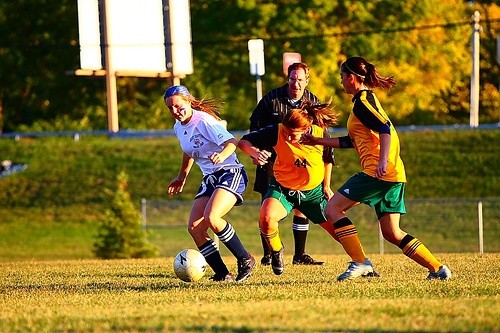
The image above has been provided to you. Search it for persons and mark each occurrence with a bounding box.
[248,61,321,266]
[164,86,256,282]
[299,56,450,280]
[239,96,342,275]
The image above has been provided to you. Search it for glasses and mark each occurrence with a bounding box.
[288,78,305,84]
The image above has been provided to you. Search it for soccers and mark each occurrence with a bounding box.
[174,249,207,281]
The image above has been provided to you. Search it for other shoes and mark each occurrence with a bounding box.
[261,254,272,265]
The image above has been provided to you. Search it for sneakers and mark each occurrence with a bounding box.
[293,254,325,265]
[209,272,236,283]
[337,257,374,281]
[425,264,452,279]
[269,241,285,275]
[235,252,255,282]
